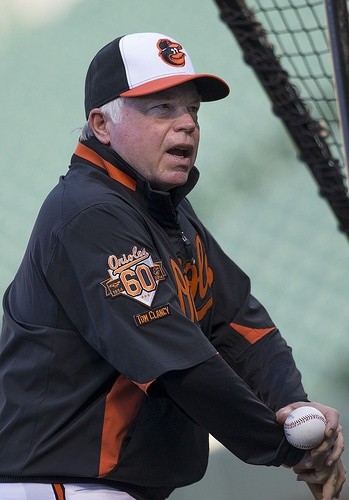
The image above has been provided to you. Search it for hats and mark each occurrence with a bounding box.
[84,33,230,121]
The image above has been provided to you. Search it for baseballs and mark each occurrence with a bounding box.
[284,407,326,449]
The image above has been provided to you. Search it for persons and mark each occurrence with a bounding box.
[0,27,348,500]
